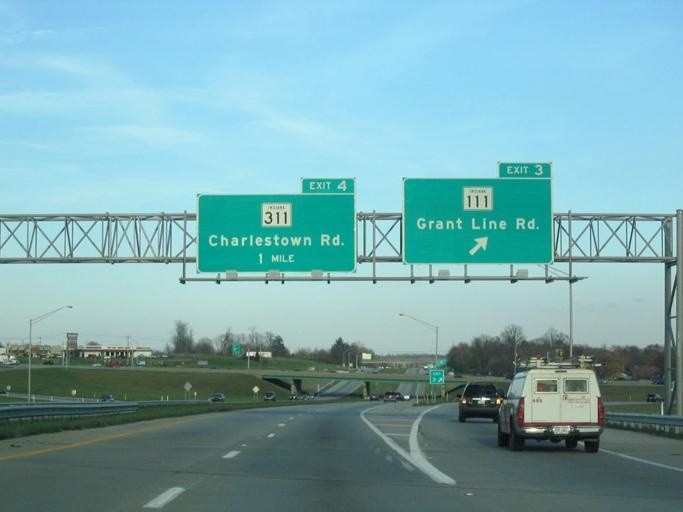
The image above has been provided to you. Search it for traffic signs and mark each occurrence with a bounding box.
[430,369,444,385]
[401,176,554,265]
[195,193,357,273]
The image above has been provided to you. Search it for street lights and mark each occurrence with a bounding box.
[341,349,372,370]
[398,313,438,402]
[27,305,72,403]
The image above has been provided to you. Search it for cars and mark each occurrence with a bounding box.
[647,393,663,402]
[264,391,275,400]
[207,391,224,401]
[96,394,113,403]
[447,369,461,376]
[288,392,314,400]
[364,392,410,401]
[652,376,664,384]
[90,358,146,368]
[494,388,506,399]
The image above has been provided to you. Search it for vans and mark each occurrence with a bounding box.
[493,348,604,453]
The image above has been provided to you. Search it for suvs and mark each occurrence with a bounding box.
[455,381,504,422]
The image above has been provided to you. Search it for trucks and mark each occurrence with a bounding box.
[0,348,19,365]
